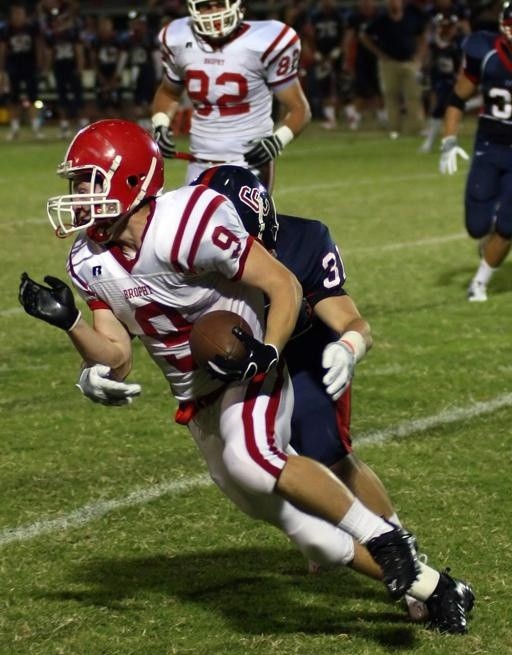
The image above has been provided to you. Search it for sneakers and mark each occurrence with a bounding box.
[429,566,477,636]
[469,278,490,302]
[367,517,420,601]
[406,594,428,621]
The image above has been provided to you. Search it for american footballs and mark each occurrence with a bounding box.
[188,310,253,375]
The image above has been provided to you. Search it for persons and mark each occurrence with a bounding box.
[87,13,128,118]
[0,8,46,141]
[362,1,433,140]
[187,165,404,538]
[150,0,313,185]
[440,1,512,303]
[42,5,89,140]
[418,11,468,154]
[120,11,161,119]
[284,0,362,133]
[19,119,474,633]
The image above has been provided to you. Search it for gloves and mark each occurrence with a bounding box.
[151,113,176,158]
[18,273,81,334]
[207,328,278,382]
[76,366,142,407]
[322,333,366,403]
[440,137,468,176]
[244,126,293,166]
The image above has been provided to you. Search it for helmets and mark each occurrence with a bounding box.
[189,0,243,39]
[189,165,279,258]
[498,3,512,40]
[46,119,164,237]
